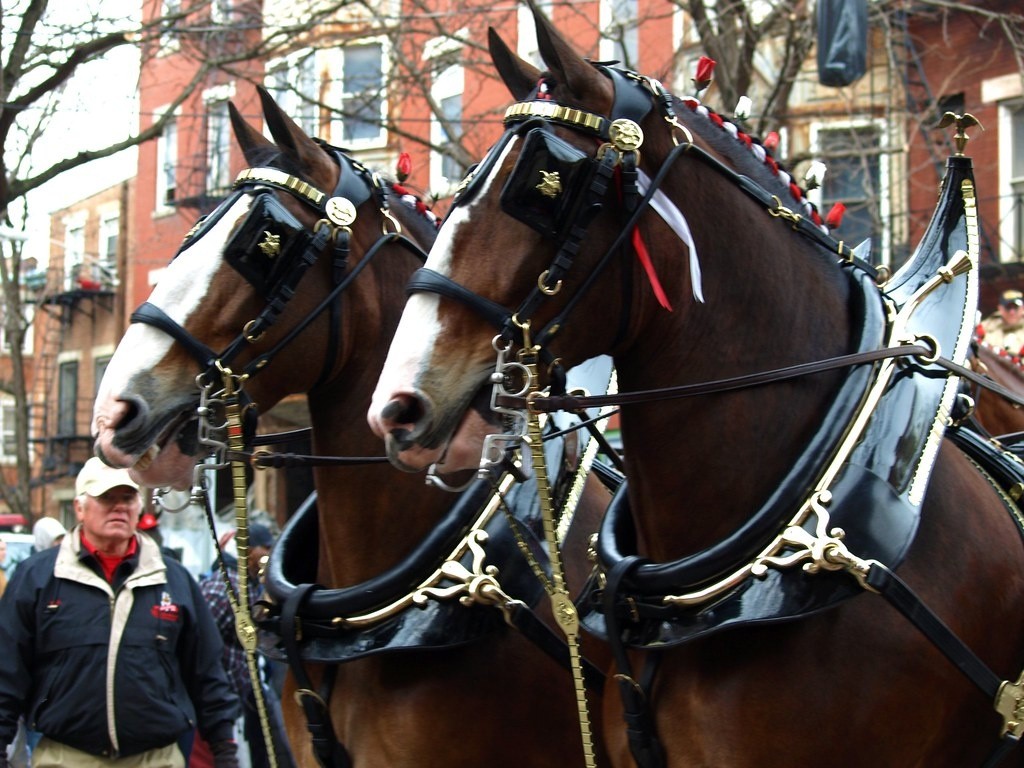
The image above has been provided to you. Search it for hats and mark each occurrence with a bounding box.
[248,524,275,548]
[76,456,140,497]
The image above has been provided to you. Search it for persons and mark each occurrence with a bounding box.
[974,290,1024,366]
[0,455,276,768]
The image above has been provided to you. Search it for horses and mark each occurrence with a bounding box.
[91,1,1024,768]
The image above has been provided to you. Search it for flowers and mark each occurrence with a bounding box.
[383,153,444,230]
[674,55,848,238]
